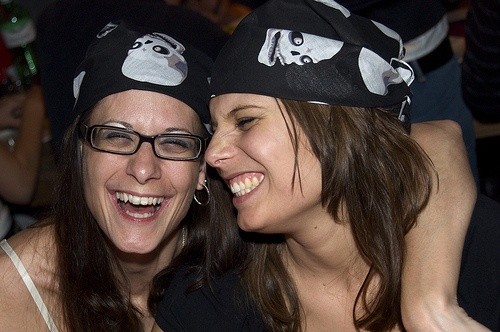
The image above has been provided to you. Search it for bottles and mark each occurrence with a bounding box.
[0,0,42,85]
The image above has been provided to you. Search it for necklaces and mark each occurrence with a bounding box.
[181,226,185,248]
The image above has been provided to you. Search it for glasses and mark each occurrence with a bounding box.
[80,122,203,161]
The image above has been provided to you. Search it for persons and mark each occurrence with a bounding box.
[0,0,500,332]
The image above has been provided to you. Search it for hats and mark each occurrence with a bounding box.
[73,18,213,135]
[207,0,415,135]
